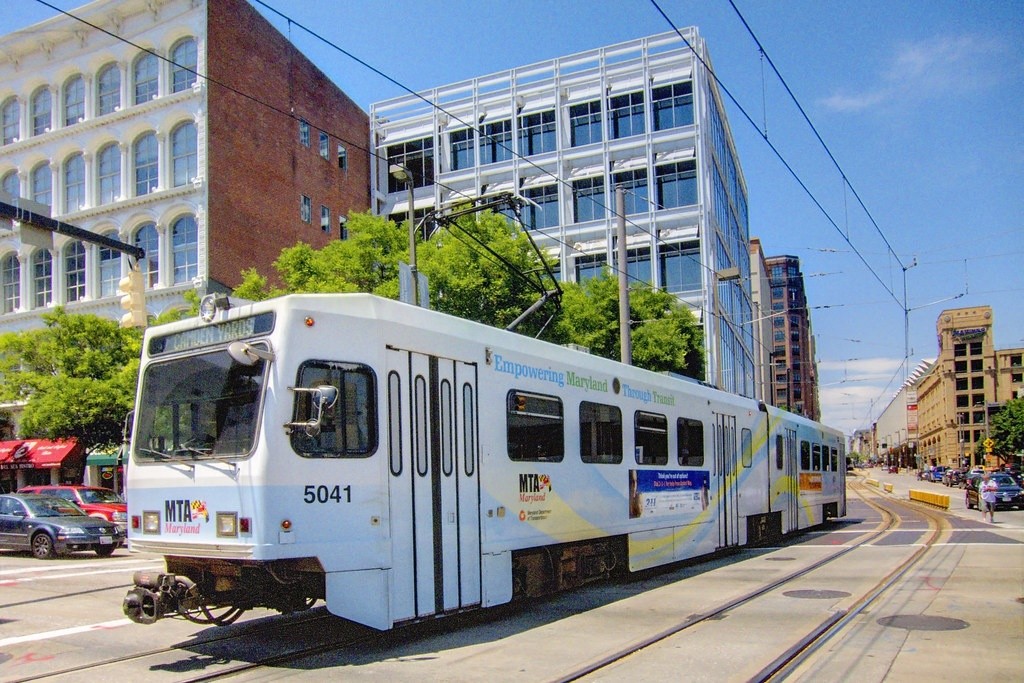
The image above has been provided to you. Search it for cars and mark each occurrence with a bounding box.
[966,473,1024,511]
[943,469,986,487]
[917,470,930,481]
[882,465,888,471]
[0,493,126,559]
[1006,470,1024,487]
[889,466,898,474]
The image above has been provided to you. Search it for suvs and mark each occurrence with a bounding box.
[17,486,127,538]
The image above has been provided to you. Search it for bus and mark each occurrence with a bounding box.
[121,290,847,633]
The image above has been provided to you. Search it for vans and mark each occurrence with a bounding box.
[929,466,951,482]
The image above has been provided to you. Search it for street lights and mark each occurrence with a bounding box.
[391,164,418,302]
[974,405,985,437]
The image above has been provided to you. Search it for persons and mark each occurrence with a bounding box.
[978,472,999,523]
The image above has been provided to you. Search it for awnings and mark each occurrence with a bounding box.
[85,448,122,466]
[0,436,79,471]
